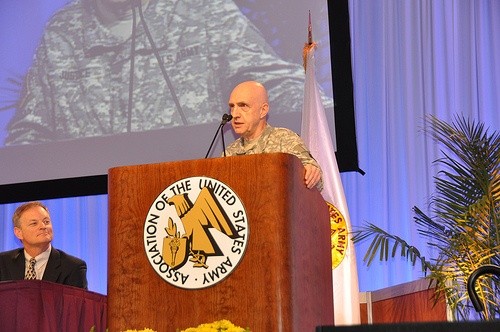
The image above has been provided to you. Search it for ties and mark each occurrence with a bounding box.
[24,257,36,280]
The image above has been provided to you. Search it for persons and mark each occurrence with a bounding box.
[3,0,336,150]
[222,81,323,192]
[0,202,88,290]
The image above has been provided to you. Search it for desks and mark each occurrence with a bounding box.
[0,280,107,332]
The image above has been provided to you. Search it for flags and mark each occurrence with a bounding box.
[300,41,360,326]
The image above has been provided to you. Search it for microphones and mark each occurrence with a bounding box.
[221,115,233,157]
[205,114,228,159]
